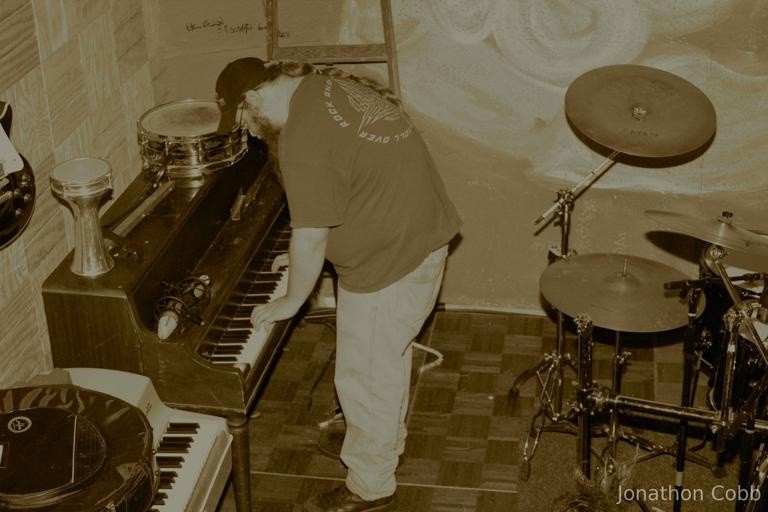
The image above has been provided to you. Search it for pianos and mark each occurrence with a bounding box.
[1,368,233,512]
[40,130,292,512]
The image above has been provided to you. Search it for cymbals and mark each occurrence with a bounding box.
[643,209,768,257]
[565,64,716,159]
[539,252,707,333]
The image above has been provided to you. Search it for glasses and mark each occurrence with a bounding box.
[237,100,250,134]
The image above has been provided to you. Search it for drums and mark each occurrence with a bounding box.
[683,262,768,377]
[136,98,248,183]
[49,156,115,279]
[710,298,768,434]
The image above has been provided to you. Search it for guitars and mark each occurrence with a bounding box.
[0,102,35,249]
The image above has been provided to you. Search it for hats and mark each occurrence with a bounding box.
[215,57,266,138]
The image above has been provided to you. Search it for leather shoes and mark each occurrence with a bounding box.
[315,430,348,460]
[302,482,398,511]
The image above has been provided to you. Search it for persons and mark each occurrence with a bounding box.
[214,56,463,511]
